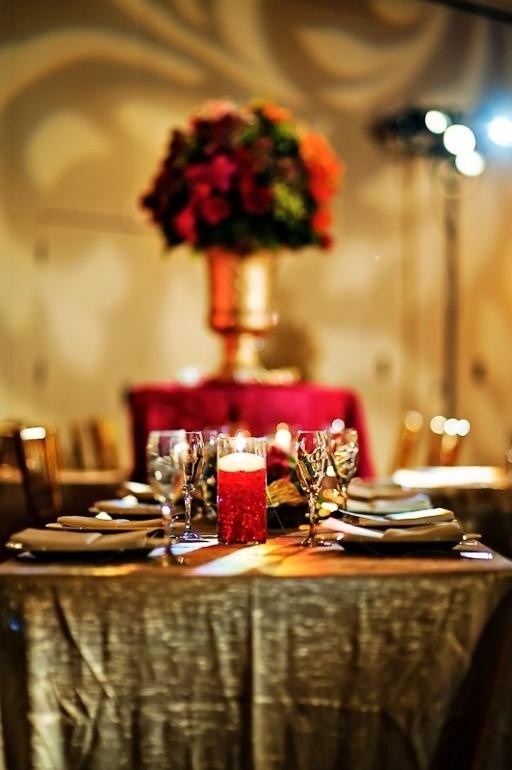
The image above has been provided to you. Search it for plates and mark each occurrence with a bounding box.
[8,481,174,563]
[327,494,465,552]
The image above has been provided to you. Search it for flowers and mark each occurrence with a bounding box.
[137,95,336,258]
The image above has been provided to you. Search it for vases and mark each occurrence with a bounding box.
[198,243,300,386]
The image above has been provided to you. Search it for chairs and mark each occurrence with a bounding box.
[12,425,64,522]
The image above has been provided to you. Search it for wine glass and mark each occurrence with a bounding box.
[174,431,206,541]
[327,428,359,511]
[294,428,333,546]
[144,428,191,568]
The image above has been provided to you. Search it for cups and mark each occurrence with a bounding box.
[216,435,270,544]
